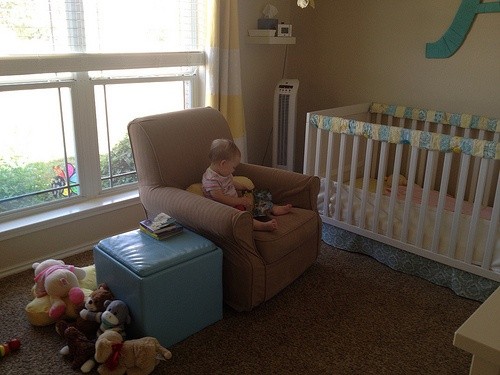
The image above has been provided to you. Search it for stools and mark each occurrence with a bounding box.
[93,227,223,347]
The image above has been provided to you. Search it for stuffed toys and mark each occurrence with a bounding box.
[25,259,172,375]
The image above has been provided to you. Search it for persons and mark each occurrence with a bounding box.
[201,139,291,231]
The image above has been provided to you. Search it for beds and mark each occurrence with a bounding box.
[304,104,500,303]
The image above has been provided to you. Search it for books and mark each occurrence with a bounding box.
[140,212,184,242]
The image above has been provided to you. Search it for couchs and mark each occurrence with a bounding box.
[127,106,322,310]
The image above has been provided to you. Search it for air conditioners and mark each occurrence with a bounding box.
[273,78,299,171]
